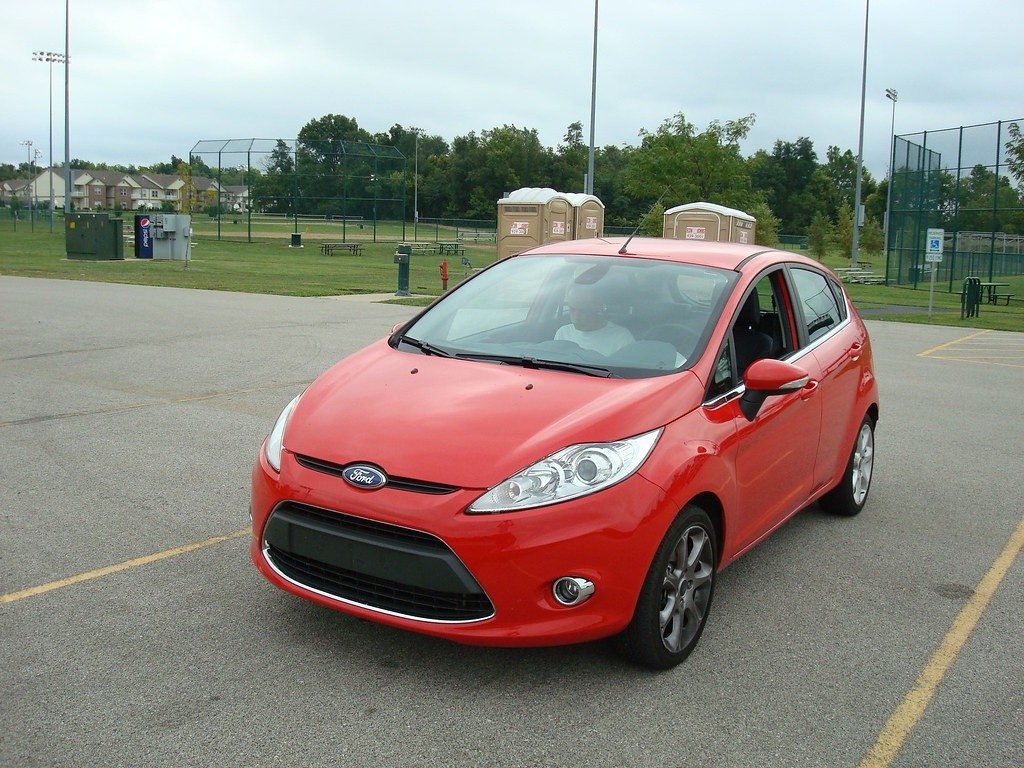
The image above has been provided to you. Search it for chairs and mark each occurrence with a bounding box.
[711,282,776,382]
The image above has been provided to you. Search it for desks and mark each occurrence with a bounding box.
[962,282,1010,304]
[321,243,362,255]
[436,242,463,254]
[404,242,432,255]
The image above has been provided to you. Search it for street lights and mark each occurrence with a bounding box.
[881,86,898,257]
[22,139,33,211]
[34,148,43,204]
[31,51,66,215]
[409,126,427,228]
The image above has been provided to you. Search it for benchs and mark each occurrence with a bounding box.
[834,268,863,280]
[394,247,423,253]
[991,294,1015,306]
[866,279,897,285]
[846,272,874,284]
[320,245,354,251]
[956,291,983,302]
[327,249,365,256]
[446,249,466,256]
[856,275,885,284]
[411,249,436,255]
[431,246,453,254]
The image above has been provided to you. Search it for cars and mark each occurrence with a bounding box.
[244,234,880,671]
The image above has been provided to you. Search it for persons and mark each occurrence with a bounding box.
[553,283,636,357]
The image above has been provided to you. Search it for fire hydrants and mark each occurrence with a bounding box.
[440,258,448,289]
